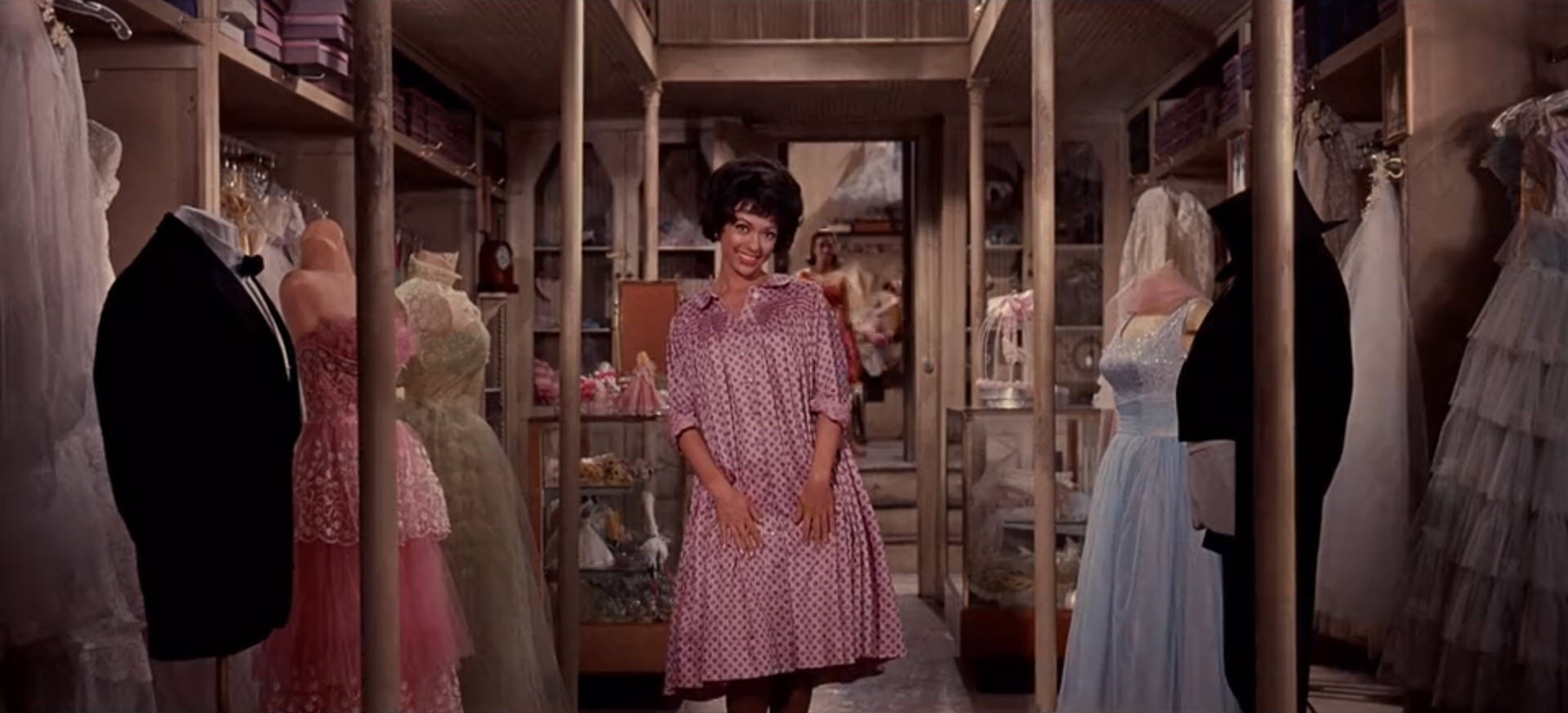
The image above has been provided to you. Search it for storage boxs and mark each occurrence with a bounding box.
[217,0,479,166]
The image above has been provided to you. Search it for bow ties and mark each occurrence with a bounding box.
[237,255,265,277]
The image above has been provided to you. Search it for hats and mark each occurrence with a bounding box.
[1207,171,1352,281]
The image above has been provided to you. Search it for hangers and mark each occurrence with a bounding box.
[222,131,298,212]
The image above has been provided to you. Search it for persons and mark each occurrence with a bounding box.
[251,219,470,713]
[1053,267,1236,713]
[791,229,873,458]
[658,156,905,713]
[391,250,572,713]
[92,205,304,662]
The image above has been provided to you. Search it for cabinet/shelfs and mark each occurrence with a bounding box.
[78,0,504,713]
[531,412,704,673]
[508,117,735,394]
[945,0,1568,713]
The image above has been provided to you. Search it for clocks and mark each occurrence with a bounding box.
[478,240,518,293]
